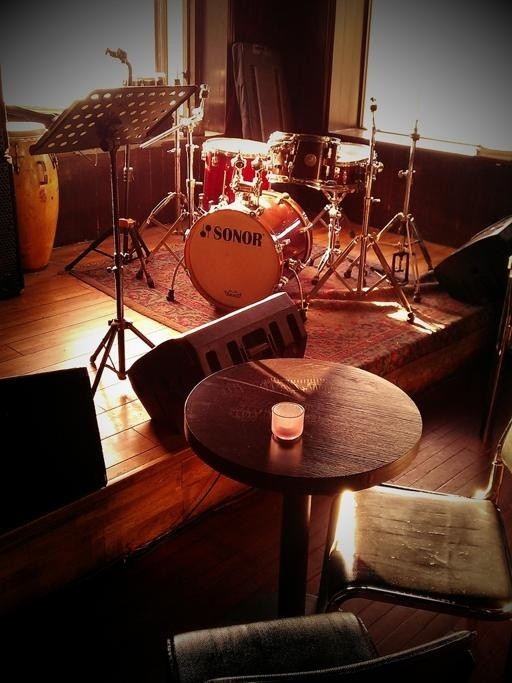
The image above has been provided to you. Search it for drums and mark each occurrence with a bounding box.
[199,137,271,216]
[7,127,60,272]
[183,188,312,314]
[262,130,340,191]
[334,142,383,196]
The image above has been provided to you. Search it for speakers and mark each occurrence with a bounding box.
[0,367,108,536]
[419,215,512,306]
[128,292,307,434]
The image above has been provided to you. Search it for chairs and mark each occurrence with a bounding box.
[166,609,476,678]
[317,417,512,620]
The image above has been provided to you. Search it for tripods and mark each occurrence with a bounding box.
[343,119,433,286]
[305,97,415,324]
[90,149,155,400]
[309,190,368,285]
[130,72,208,302]
[64,45,155,289]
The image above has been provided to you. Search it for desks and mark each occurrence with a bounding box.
[183,356,425,618]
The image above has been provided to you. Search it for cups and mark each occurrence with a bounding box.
[272,401,305,439]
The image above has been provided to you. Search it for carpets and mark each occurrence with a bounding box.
[67,229,481,380]
